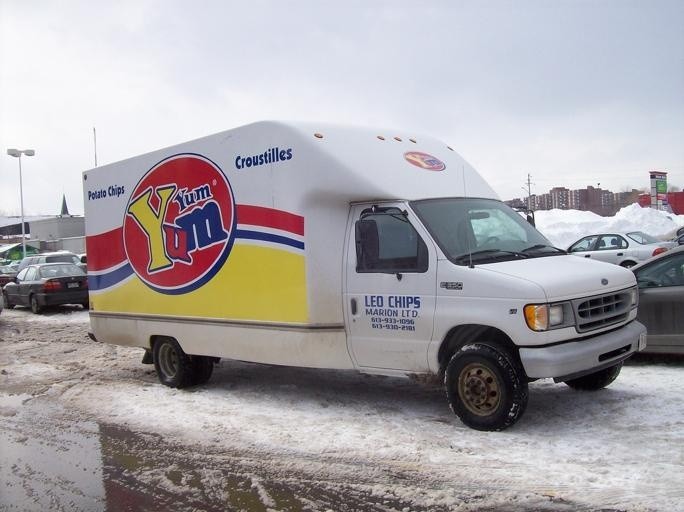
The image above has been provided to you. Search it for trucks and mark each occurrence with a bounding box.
[82,120,647,432]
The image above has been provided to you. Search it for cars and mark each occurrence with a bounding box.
[0,250,89,313]
[664,226,684,245]
[627,245,683,357]
[563,229,679,267]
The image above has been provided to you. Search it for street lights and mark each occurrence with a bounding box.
[521,186,536,214]
[6,148,37,259]
